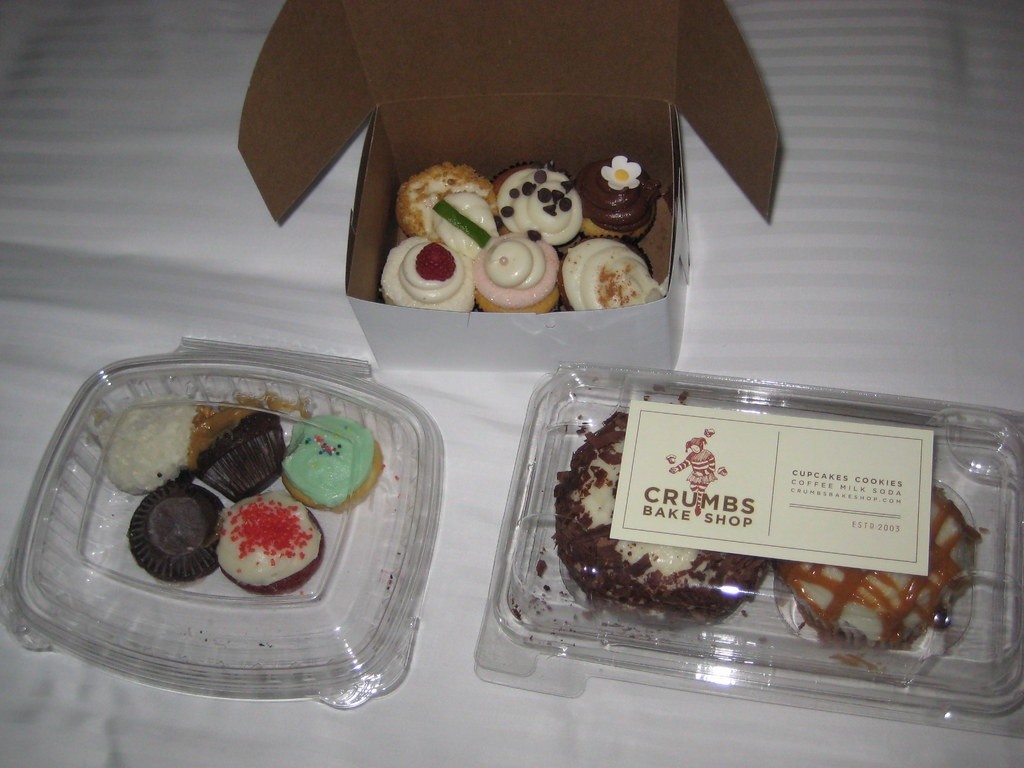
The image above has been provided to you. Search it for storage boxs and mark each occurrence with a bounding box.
[237,0,781,372]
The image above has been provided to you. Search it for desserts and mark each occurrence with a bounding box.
[381,156,662,312]
[553,409,983,650]
[106,401,383,594]
